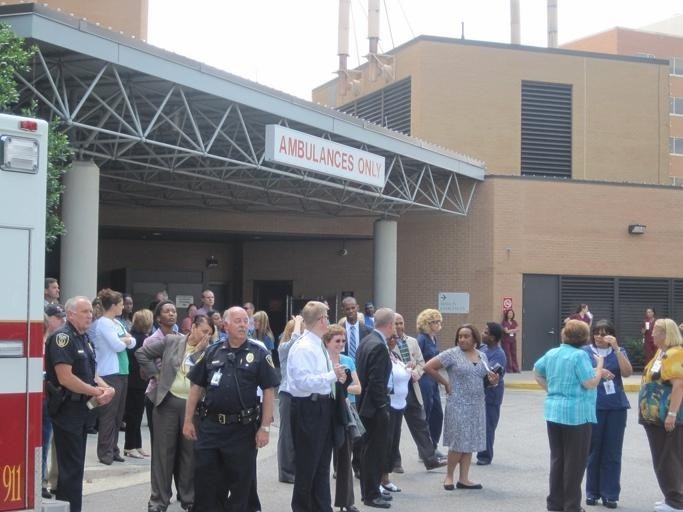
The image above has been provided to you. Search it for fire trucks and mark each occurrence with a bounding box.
[1,113,49,512]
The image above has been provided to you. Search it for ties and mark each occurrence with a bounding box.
[321,341,336,400]
[348,326,356,362]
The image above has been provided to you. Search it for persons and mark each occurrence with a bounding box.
[563,302,592,345]
[639,307,658,367]
[474,324,508,465]
[531,320,603,511]
[677,321,682,330]
[41,278,443,511]
[423,323,499,490]
[637,318,683,511]
[501,309,520,373]
[580,318,633,507]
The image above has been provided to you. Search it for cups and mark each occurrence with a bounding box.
[586,312,593,319]
[644,322,649,330]
[86,395,100,409]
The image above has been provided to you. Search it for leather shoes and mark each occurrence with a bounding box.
[100,448,150,465]
[418,454,491,490]
[333,467,404,508]
[548,497,683,512]
[42,488,51,498]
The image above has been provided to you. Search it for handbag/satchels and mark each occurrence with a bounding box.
[484,362,505,388]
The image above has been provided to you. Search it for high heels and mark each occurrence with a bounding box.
[341,505,360,512]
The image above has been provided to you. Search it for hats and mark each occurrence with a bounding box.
[45,303,66,317]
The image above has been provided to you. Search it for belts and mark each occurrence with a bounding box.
[206,410,252,425]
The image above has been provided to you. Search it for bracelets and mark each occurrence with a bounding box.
[667,411,677,416]
[614,348,621,355]
